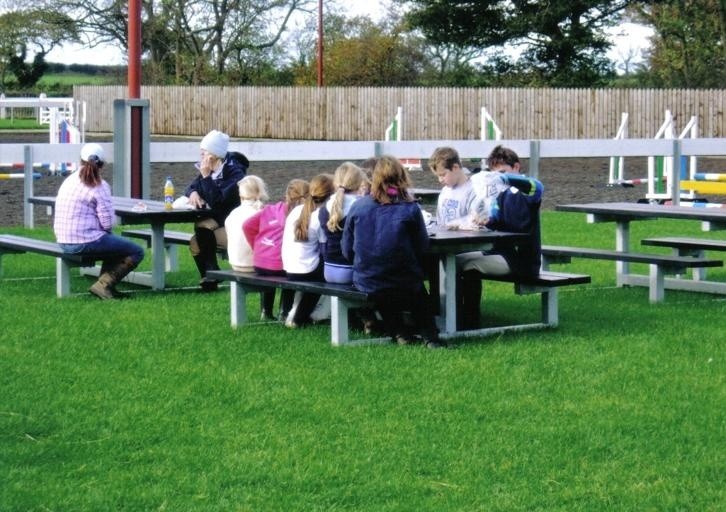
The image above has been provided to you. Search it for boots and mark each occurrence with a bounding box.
[89,256,136,298]
[192,227,223,292]
[462,269,482,329]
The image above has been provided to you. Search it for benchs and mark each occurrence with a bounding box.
[538,244,723,303]
[0,233,95,298]
[121,229,228,273]
[488,269,591,327]
[204,270,408,347]
[639,238,725,282]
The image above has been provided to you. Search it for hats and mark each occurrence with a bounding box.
[200,130,229,158]
[80,143,104,162]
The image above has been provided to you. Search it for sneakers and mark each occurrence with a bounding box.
[262,311,440,347]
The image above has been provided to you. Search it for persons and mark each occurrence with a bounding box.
[183,129,249,292]
[53,142,145,301]
[224,145,545,349]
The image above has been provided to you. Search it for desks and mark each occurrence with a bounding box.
[555,202,726,298]
[28,195,217,292]
[331,224,559,348]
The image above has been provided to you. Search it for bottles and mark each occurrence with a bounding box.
[163,176,174,210]
